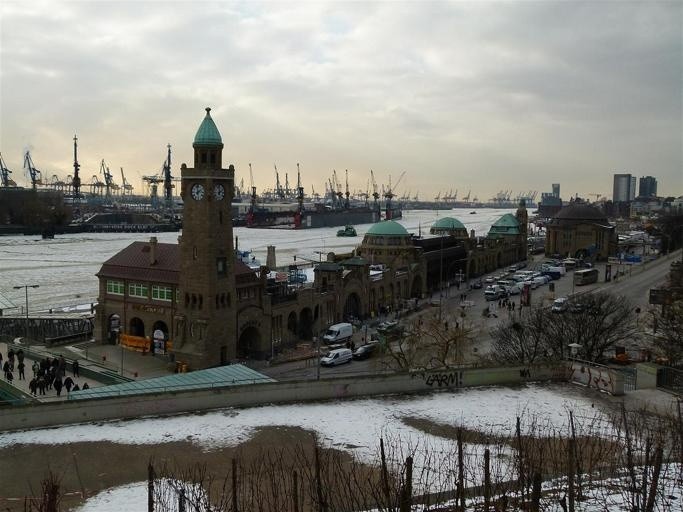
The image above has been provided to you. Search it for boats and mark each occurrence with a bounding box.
[336,222,357,237]
[468,210,477,214]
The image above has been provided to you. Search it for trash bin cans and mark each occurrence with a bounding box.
[177,361,189,373]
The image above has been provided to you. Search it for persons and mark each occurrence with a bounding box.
[460,292,464,304]
[464,292,467,302]
[498,296,515,311]
[0,348,89,395]
[369,303,392,320]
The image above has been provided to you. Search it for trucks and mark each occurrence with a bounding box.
[549,297,569,314]
[320,320,404,368]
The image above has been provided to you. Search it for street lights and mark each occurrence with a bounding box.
[312,291,328,380]
[566,342,582,390]
[313,250,329,293]
[13,284,39,354]
[78,313,92,363]
[108,313,124,377]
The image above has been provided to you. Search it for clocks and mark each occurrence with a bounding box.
[212,183,226,203]
[189,183,203,201]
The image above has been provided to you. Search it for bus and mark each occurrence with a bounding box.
[572,269,600,285]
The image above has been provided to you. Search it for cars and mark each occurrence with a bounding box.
[469,255,581,302]
